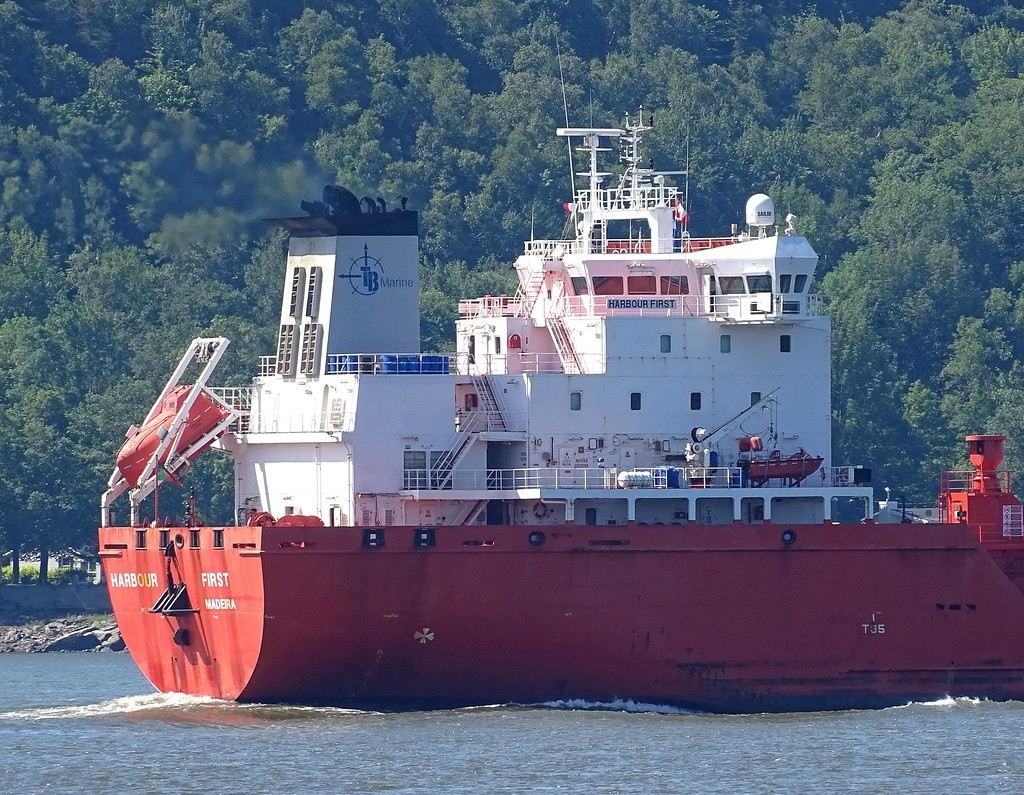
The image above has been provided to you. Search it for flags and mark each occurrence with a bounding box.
[564,203,577,216]
[675,200,691,224]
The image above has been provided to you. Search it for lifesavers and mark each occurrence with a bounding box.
[533,502,548,518]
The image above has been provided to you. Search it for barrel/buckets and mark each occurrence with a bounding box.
[422,356,430,374]
[710,450,718,467]
[339,357,347,374]
[383,356,397,374]
[671,229,681,252]
[350,356,358,374]
[484,295,491,309]
[444,357,449,374]
[397,356,407,374]
[654,467,680,489]
[431,356,438,374]
[407,356,420,374]
[329,356,337,374]
[465,394,477,410]
[499,293,508,309]
[733,473,741,488]
[439,357,443,374]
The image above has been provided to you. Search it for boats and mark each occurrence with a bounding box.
[738,450,824,482]
[98,42,1024,713]
